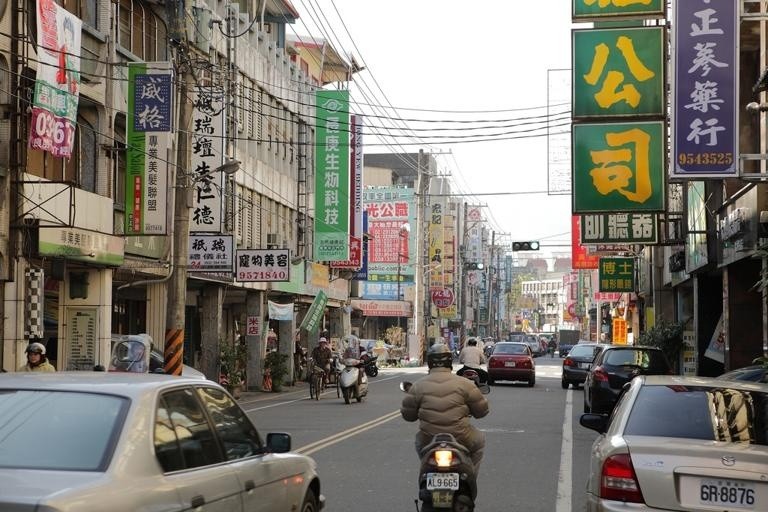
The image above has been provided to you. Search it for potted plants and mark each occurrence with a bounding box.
[216,334,247,399]
[264,352,289,392]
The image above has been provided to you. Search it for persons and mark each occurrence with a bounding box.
[117,331,158,372]
[311,334,332,388]
[454,337,490,388]
[342,339,368,386]
[547,337,557,359]
[16,341,57,373]
[383,335,398,365]
[399,341,491,479]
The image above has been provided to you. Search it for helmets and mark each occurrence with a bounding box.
[468,339,477,344]
[429,343,452,368]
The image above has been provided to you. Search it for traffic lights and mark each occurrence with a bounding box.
[511,240,540,251]
[463,261,483,271]
[546,302,555,306]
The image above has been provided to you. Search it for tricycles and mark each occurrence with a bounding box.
[307,357,342,401]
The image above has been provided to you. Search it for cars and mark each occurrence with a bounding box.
[504,329,556,356]
[0,373,325,512]
[579,375,767,512]
[485,341,538,387]
[359,338,400,363]
[560,343,603,389]
[714,365,768,383]
[582,345,673,418]
[110,332,209,382]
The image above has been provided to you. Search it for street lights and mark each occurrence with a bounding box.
[162,156,243,377]
[397,224,411,302]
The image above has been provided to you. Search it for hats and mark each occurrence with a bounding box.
[318,337,327,343]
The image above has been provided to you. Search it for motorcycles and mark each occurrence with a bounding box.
[358,347,380,377]
[337,353,369,404]
[483,344,492,358]
[397,379,490,511]
[455,349,487,391]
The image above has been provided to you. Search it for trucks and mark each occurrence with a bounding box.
[557,329,580,358]
